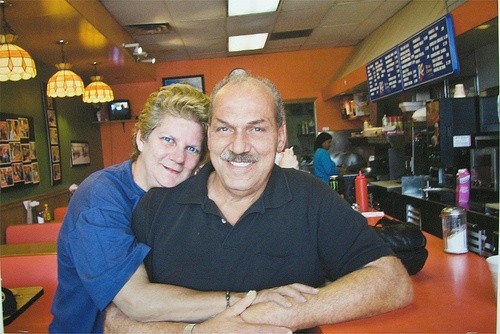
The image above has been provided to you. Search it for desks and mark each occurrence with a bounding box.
[316,209,496,334]
[3,286,44,326]
[0,242,57,257]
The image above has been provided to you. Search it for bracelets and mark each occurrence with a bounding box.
[225,291,231,309]
[182,324,197,334]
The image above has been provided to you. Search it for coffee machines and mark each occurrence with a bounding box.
[43,203,51,222]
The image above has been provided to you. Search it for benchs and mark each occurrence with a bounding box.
[54,207,67,220]
[0,255,58,334]
[6,224,63,245]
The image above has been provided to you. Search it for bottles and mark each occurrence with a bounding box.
[343,100,349,118]
[453,84,467,98]
[383,113,404,130]
[363,120,369,130]
[297,119,315,136]
[486,255,498,296]
[354,170,369,212]
[439,207,467,253]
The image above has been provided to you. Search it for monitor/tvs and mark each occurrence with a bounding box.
[108,99,132,119]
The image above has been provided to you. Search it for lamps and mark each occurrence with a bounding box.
[47,39,84,97]
[126,43,155,64]
[0,0,37,81]
[83,62,114,103]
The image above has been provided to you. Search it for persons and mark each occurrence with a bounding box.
[312,132,346,188]
[104,73,414,334]
[47,82,318,334]
